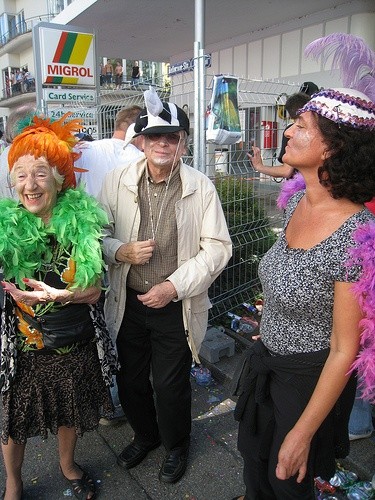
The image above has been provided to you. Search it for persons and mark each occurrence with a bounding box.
[130,61,142,91]
[0,111,115,500]
[115,61,123,91]
[98,59,113,88]
[0,104,143,427]
[230,87,375,500]
[5,68,35,98]
[246,81,375,440]
[95,85,232,485]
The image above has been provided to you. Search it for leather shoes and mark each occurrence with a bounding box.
[159,447,189,484]
[117,439,157,469]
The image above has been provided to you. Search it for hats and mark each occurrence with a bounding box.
[284,81,320,118]
[295,33,375,131]
[8,111,89,189]
[134,85,190,135]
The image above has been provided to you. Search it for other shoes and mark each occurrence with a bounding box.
[231,495,245,500]
[99,415,127,425]
[349,426,373,440]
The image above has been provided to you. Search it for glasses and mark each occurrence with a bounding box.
[148,132,180,144]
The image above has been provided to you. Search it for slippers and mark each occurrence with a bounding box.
[1,480,23,500]
[60,462,96,500]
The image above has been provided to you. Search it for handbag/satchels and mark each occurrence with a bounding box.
[205,75,242,145]
[39,303,96,348]
